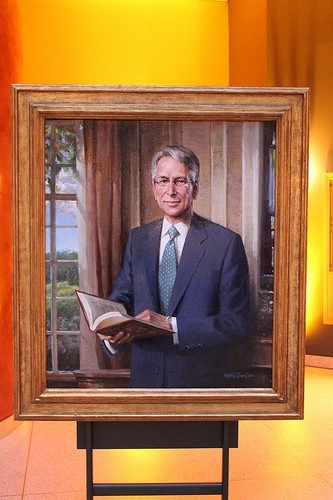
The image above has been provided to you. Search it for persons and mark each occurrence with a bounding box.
[97,144,250,388]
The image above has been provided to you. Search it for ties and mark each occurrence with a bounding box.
[158,226,179,312]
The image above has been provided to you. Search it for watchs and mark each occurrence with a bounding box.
[167,315,173,329]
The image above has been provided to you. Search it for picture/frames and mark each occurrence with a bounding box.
[9,84,311,422]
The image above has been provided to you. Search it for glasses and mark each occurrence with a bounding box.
[155,177,193,187]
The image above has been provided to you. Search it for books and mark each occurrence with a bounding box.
[75,288,174,343]
